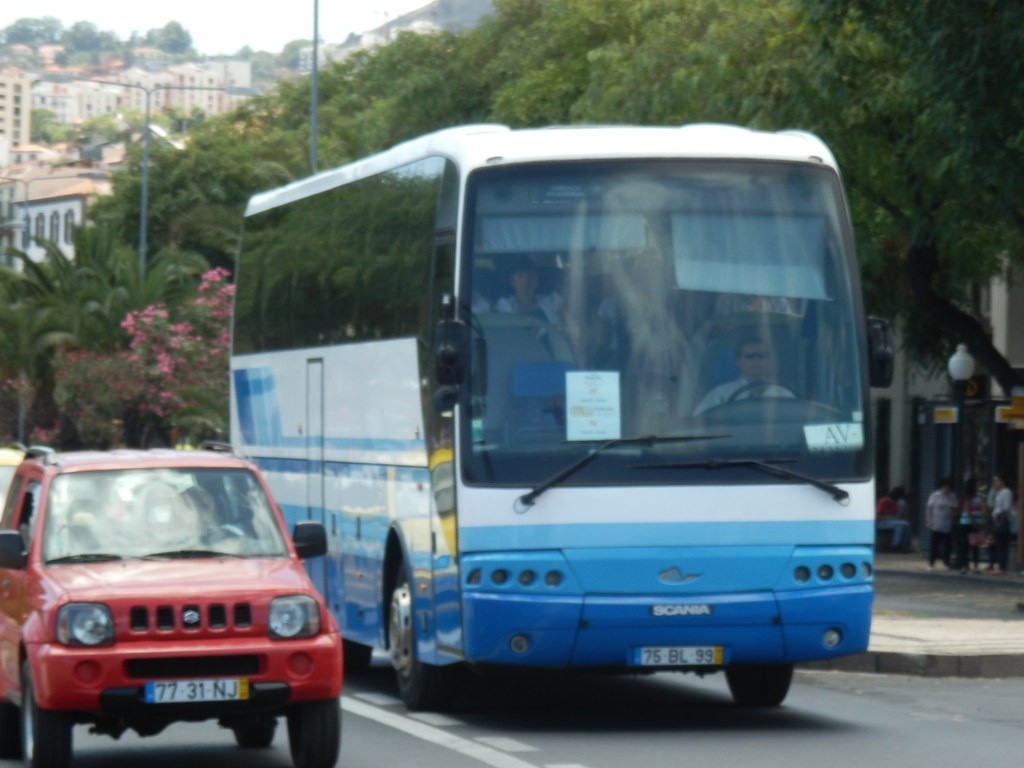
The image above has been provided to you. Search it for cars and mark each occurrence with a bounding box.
[1,442,29,523]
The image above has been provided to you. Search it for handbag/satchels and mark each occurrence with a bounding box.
[969,533,988,545]
[996,511,1017,541]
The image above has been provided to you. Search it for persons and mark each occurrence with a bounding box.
[879,470,1019,576]
[456,254,798,426]
[53,482,242,557]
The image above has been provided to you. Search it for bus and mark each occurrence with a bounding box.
[224,123,892,714]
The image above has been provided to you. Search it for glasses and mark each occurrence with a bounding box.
[739,351,765,358]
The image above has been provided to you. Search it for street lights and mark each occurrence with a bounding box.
[37,69,263,294]
[941,342,977,569]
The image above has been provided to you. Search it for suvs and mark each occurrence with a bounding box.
[0,444,344,768]
